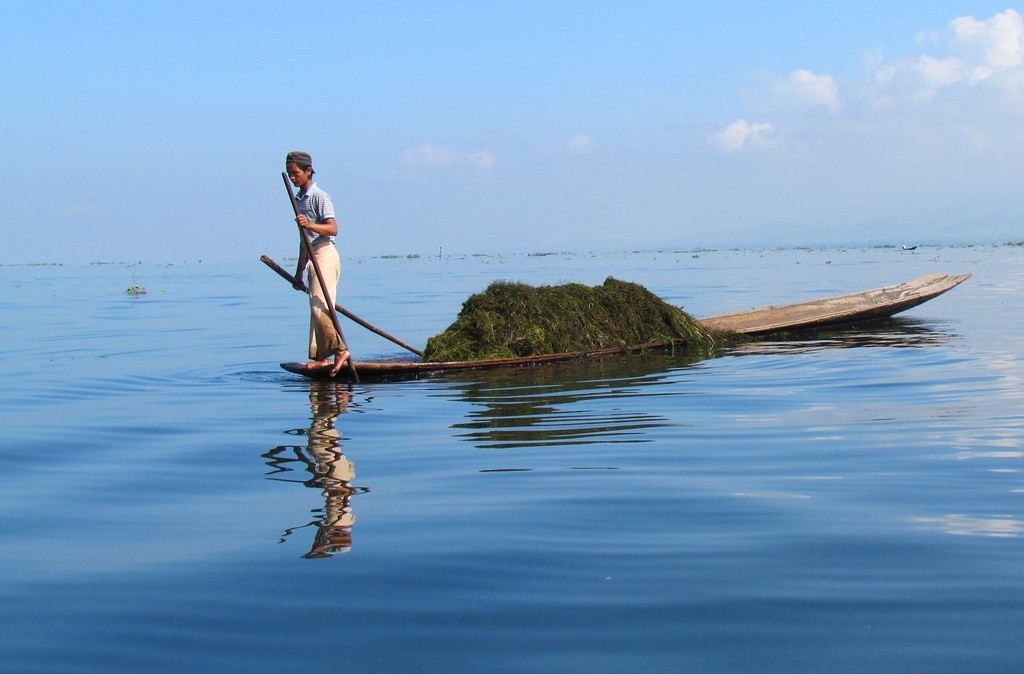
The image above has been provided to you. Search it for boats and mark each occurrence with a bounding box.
[901,244,917,250]
[278,270,973,383]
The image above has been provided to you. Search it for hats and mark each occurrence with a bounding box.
[286,151,315,174]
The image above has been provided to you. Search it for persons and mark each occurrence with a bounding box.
[286,152,349,377]
